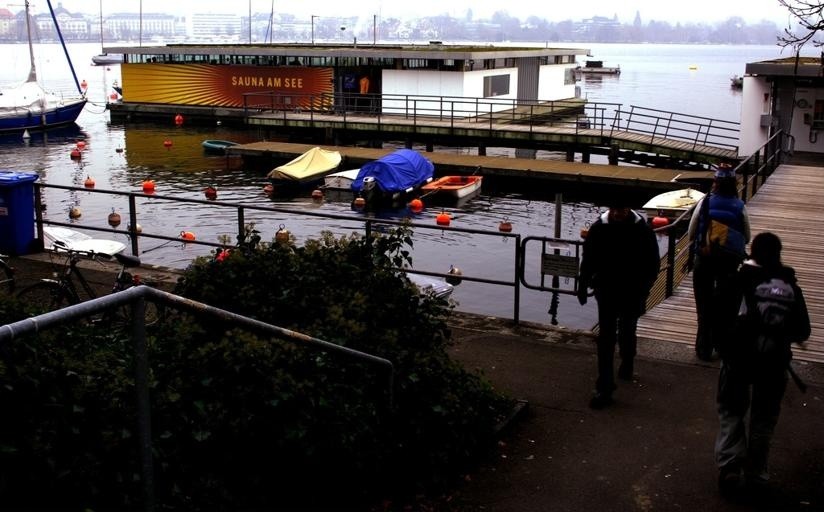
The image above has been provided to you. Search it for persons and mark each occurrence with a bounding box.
[686,163,751,362]
[577,187,661,409]
[703,231,812,504]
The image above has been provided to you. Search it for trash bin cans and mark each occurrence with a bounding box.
[0,170,40,257]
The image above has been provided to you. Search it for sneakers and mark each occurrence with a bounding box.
[695,349,722,361]
[588,359,634,410]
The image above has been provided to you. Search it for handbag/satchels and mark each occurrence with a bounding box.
[696,218,746,262]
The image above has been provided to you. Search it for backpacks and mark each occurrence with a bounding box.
[742,267,791,352]
